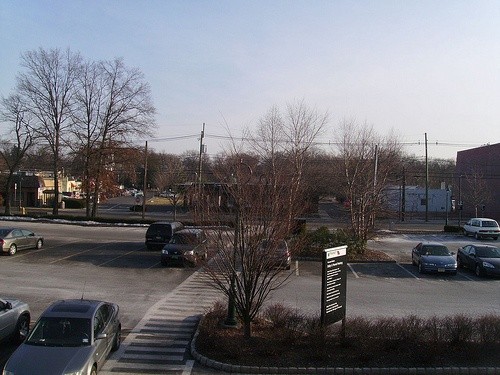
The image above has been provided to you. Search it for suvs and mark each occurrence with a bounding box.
[258,238,291,270]
[161,228,210,268]
[145,222,186,248]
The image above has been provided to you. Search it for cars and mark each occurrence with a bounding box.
[0,298,31,343]
[412,242,457,276]
[127,188,179,198]
[463,218,500,240]
[0,227,44,256]
[2,299,122,375]
[457,244,500,278]
